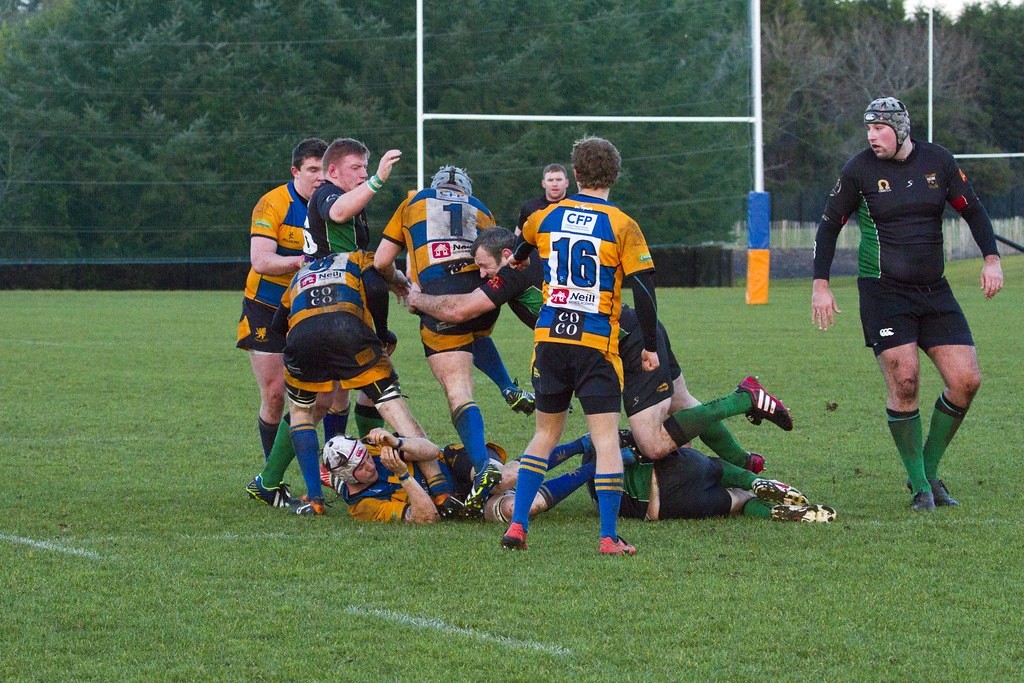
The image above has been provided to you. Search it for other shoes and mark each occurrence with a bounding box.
[769,505,836,525]
[753,478,809,506]
[585,431,633,447]
[631,445,656,463]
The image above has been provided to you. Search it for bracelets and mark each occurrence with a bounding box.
[397,472,413,485]
[397,438,405,448]
[296,255,305,269]
[366,174,386,192]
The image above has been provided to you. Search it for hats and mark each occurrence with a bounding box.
[323,434,367,486]
[864,97,910,146]
[430,164,473,196]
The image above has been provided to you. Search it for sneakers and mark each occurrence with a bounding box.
[290,496,326,517]
[744,452,767,474]
[437,493,463,519]
[502,522,529,550]
[503,379,536,417]
[246,474,301,509]
[907,479,956,505]
[320,462,332,488]
[911,489,935,511]
[599,536,636,556]
[464,460,502,518]
[735,375,793,432]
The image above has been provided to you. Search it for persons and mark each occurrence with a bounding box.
[500,136,660,554]
[236,137,837,524]
[810,96,1003,511]
